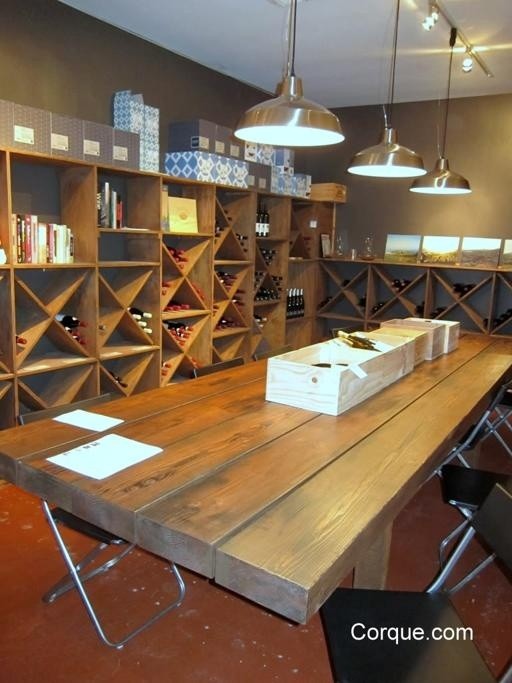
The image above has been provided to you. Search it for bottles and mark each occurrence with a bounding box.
[254,271,284,302]
[97,323,108,333]
[108,371,129,389]
[254,314,269,329]
[161,205,251,381]
[358,232,376,259]
[334,329,386,353]
[14,334,27,346]
[127,307,153,337]
[256,201,271,238]
[56,306,90,349]
[303,235,312,251]
[259,248,279,266]
[317,273,512,334]
[289,240,294,246]
[286,287,305,319]
[310,363,348,368]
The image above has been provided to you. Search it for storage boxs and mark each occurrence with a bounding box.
[262,315,465,416]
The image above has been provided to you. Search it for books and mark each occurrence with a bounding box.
[95,176,125,230]
[9,207,76,264]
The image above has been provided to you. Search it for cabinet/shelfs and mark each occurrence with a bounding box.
[0,142,337,439]
[314,252,511,347]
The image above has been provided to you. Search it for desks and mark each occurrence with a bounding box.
[0,328,510,631]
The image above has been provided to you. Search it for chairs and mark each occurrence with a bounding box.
[251,343,299,363]
[191,353,246,380]
[14,387,190,649]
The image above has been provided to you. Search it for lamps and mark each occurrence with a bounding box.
[344,0,430,178]
[418,0,493,80]
[401,25,473,196]
[233,0,347,150]
